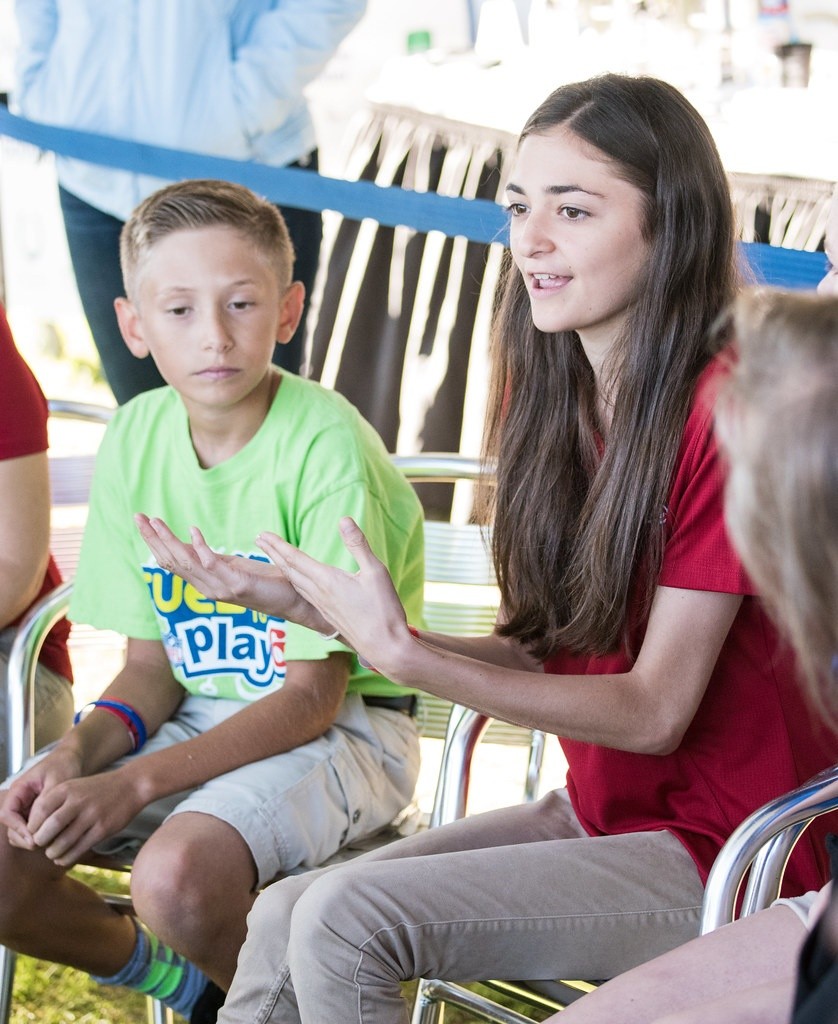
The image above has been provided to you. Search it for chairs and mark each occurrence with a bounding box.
[0,453,838,1024]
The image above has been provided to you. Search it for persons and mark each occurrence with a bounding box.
[0,0,838,1024]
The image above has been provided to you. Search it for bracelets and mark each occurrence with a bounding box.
[315,630,340,643]
[72,697,147,755]
[357,624,420,674]
